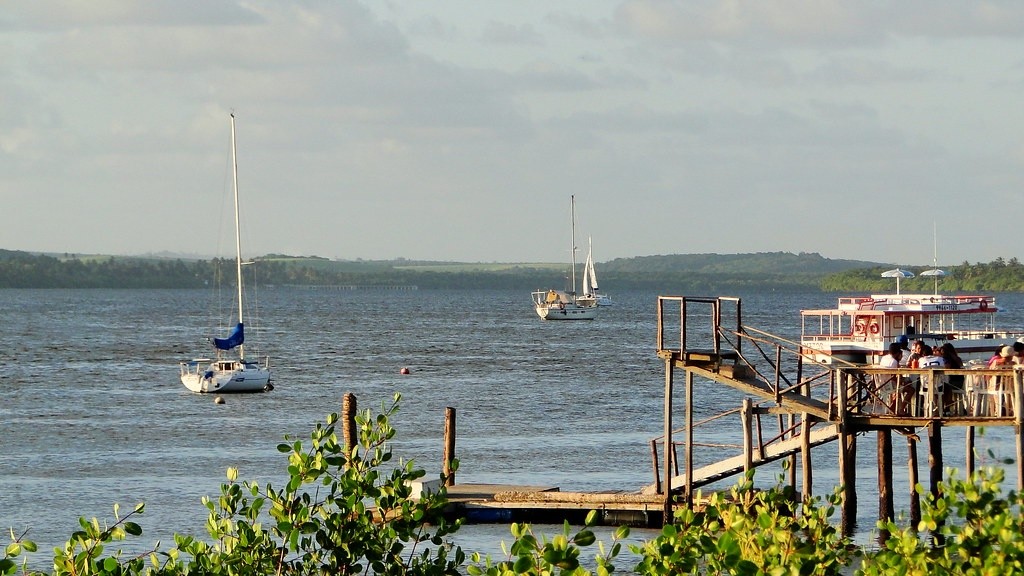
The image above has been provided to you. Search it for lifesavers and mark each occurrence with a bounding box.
[979,300,988,311]
[855,323,865,334]
[870,323,879,334]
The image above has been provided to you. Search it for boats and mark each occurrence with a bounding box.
[800,305,1024,365]
[838,259,1003,313]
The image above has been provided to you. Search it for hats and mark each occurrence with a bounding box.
[995,344,1007,355]
[896,335,907,343]
[1017,337,1024,344]
[999,346,1018,358]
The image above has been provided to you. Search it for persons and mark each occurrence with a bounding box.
[559,301,564,310]
[878,334,1024,416]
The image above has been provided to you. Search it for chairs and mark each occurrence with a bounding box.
[872,359,1015,418]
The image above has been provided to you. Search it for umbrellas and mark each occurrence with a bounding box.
[920,269,951,295]
[881,267,915,295]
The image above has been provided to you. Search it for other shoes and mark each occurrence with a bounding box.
[898,413,913,417]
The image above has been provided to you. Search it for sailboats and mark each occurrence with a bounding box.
[175,110,273,394]
[531,193,613,321]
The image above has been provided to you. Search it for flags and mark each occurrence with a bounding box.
[214,323,244,350]
